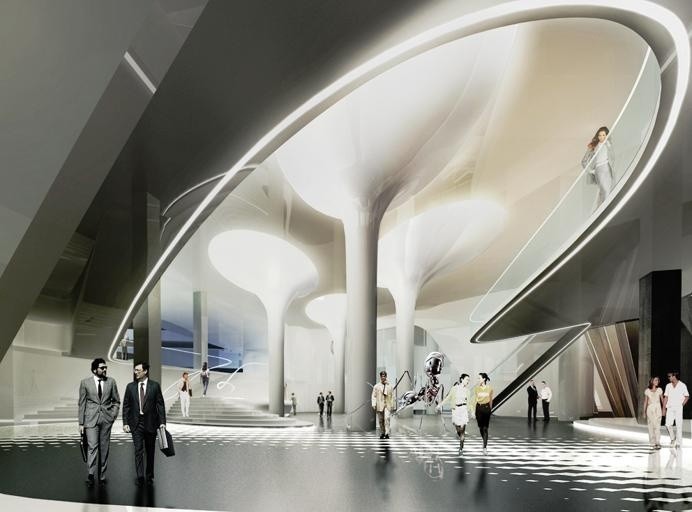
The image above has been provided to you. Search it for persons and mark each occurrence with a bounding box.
[290,393,298,415]
[371,371,395,439]
[199,362,210,397]
[325,391,334,417]
[643,376,664,454]
[177,372,191,418]
[317,392,325,417]
[122,362,166,487]
[538,381,552,423]
[641,449,692,512]
[471,372,494,455]
[662,370,689,450]
[526,380,540,422]
[78,358,121,488]
[435,374,473,456]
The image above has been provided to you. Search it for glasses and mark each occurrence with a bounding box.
[97,365,108,370]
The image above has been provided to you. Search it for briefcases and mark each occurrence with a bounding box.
[157,427,175,457]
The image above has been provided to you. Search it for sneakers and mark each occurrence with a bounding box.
[670,436,676,448]
[379,434,389,439]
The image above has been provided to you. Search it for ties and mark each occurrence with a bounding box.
[140,383,145,411]
[98,379,102,400]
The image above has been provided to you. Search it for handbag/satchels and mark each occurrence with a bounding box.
[189,389,192,396]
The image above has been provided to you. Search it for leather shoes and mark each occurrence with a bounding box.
[136,477,144,485]
[99,480,105,488]
[86,479,94,487]
[147,477,153,485]
